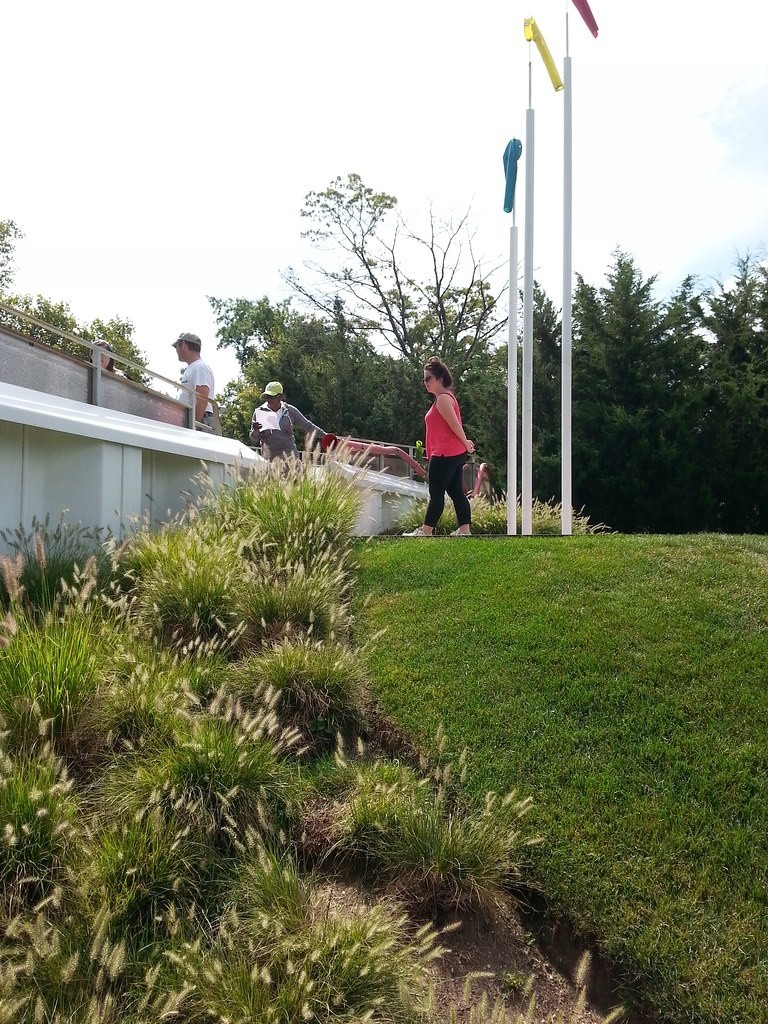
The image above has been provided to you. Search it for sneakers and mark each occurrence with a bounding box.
[402,527,433,536]
[450,527,473,536]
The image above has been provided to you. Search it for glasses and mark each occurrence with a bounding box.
[422,375,432,384]
[264,395,282,400]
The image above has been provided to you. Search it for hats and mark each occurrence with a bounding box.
[171,333,201,348]
[262,382,283,396]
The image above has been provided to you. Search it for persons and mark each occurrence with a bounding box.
[171,332,217,436]
[400,356,476,536]
[249,381,329,480]
[89,339,133,380]
